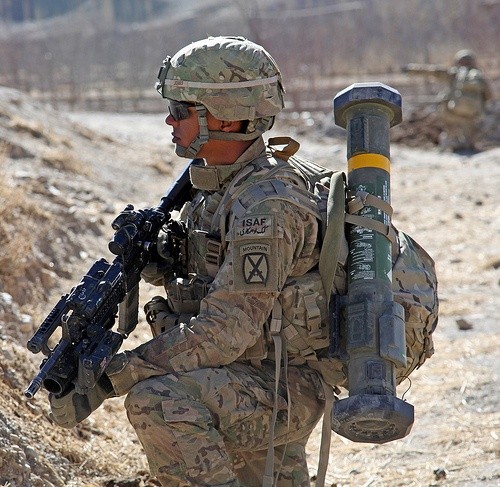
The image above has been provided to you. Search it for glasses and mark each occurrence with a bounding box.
[168,100,204,120]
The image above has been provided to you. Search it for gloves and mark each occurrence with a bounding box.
[48,371,114,429]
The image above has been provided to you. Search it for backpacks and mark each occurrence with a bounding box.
[232,156,439,394]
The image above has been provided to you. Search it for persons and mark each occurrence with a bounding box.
[401,49,492,151]
[48,36,325,487]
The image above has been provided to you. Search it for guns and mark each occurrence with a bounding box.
[24,157,206,399]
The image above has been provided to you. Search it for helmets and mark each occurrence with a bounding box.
[154,35,285,120]
